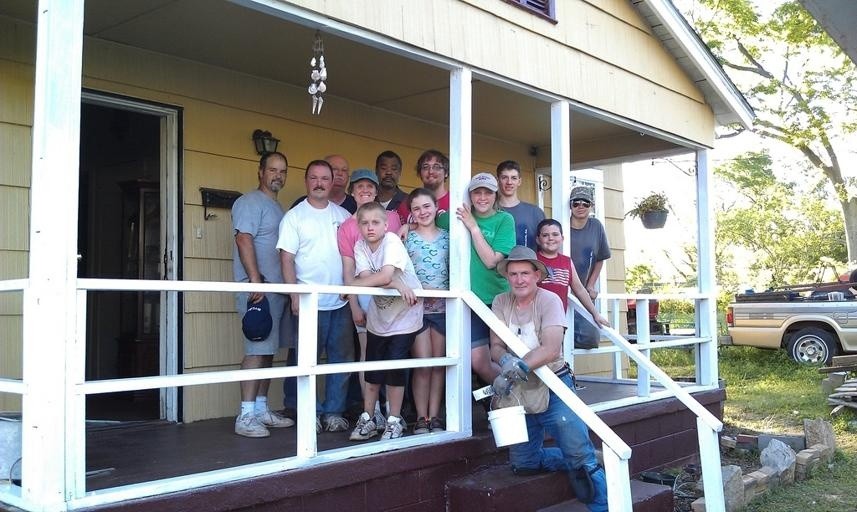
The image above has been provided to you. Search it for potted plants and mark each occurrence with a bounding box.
[623,190,678,229]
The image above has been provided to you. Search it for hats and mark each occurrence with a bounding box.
[569,184,594,207]
[239,295,273,342]
[466,171,499,194]
[495,243,549,285]
[348,168,380,187]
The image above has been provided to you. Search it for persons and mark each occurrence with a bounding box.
[488,245,608,512]
[233,146,612,444]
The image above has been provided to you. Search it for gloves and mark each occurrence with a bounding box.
[490,373,516,400]
[496,352,533,386]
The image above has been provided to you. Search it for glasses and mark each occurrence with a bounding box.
[569,199,592,209]
[420,162,445,172]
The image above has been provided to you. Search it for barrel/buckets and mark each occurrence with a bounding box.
[488,391,531,448]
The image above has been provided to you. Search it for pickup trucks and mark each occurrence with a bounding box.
[725,282,856,366]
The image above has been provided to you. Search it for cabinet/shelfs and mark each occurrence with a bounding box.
[115,180,160,404]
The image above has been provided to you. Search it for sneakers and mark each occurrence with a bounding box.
[323,413,350,433]
[316,416,322,435]
[428,416,445,433]
[374,409,387,429]
[254,407,296,429]
[379,413,408,441]
[349,410,377,442]
[412,416,430,435]
[232,413,270,439]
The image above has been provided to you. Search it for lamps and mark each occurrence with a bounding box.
[254,130,282,156]
